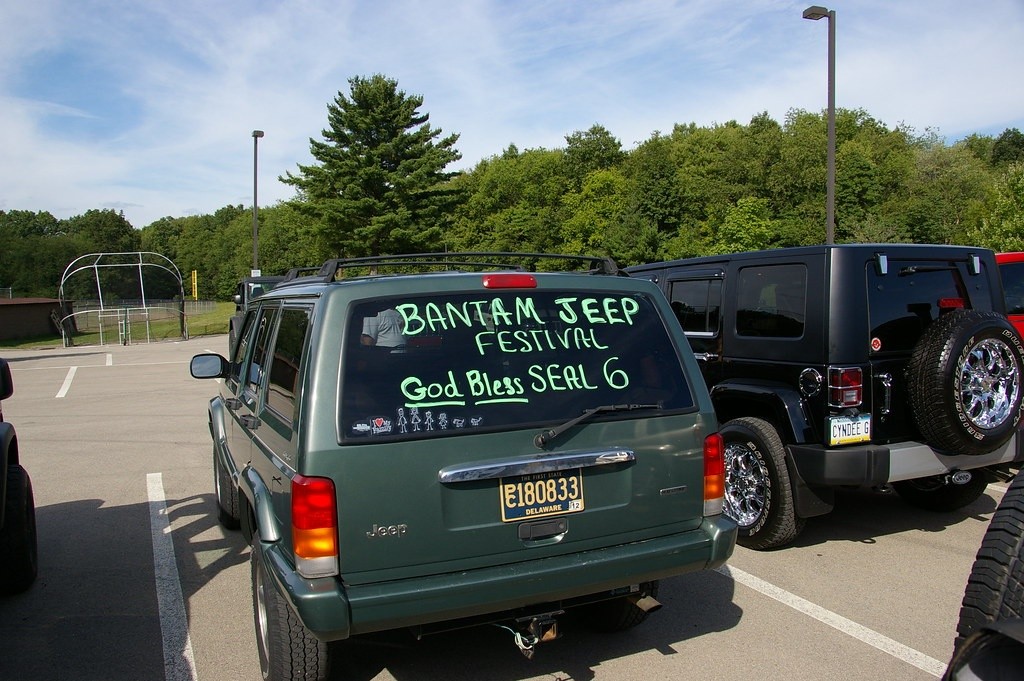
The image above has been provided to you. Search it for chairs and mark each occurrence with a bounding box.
[433,319,495,395]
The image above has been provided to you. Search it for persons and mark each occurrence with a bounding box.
[361,309,412,415]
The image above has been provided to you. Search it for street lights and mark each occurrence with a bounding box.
[251,129,265,278]
[802,5,836,246]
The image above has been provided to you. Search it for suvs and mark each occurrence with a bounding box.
[939,250,1024,340]
[192,252,739,681]
[613,242,1024,554]
[0,358,40,598]
